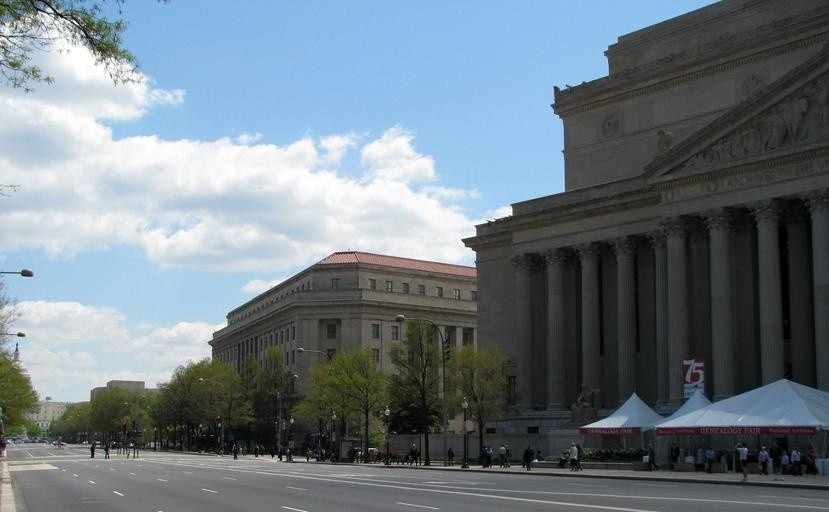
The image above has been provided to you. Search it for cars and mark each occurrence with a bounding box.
[4,435,67,446]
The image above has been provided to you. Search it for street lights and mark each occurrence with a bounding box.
[276,374,298,457]
[288,314,470,472]
[196,375,224,389]
[0,331,26,338]
[119,421,223,452]
[0,267,35,279]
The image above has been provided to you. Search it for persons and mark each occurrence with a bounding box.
[505,444,512,469]
[482,444,492,468]
[558,442,584,471]
[231,444,335,461]
[104,441,110,459]
[534,448,544,461]
[648,443,658,471]
[89,439,96,458]
[448,447,455,466]
[736,442,749,482]
[791,447,803,476]
[524,444,533,471]
[780,450,789,475]
[759,445,769,475]
[410,444,418,466]
[671,442,679,470]
[806,443,819,475]
[705,444,715,474]
[406,453,412,465]
[350,447,370,463]
[499,444,505,468]
